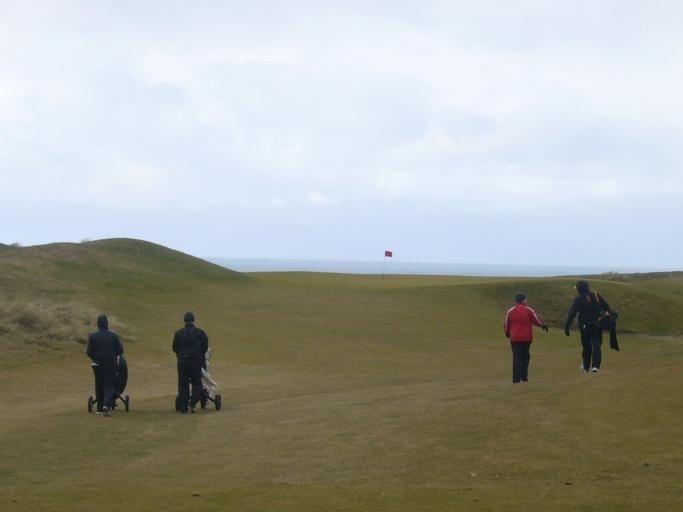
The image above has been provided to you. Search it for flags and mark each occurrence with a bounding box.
[385,251,392,257]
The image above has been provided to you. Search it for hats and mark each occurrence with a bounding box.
[184,312,194,322]
[573,280,589,289]
[97,314,108,329]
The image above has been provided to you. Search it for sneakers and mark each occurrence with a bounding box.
[513,379,528,382]
[182,408,197,414]
[580,364,601,374]
[96,406,111,416]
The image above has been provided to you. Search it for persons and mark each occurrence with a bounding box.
[87,314,124,416]
[565,280,610,373]
[504,292,549,383]
[172,312,208,413]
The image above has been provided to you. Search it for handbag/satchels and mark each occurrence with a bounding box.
[596,310,618,330]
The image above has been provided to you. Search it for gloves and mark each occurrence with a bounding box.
[543,325,548,333]
[565,329,569,336]
[506,333,510,337]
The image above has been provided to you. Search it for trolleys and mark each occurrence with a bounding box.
[175,363,221,413]
[88,356,131,415]
